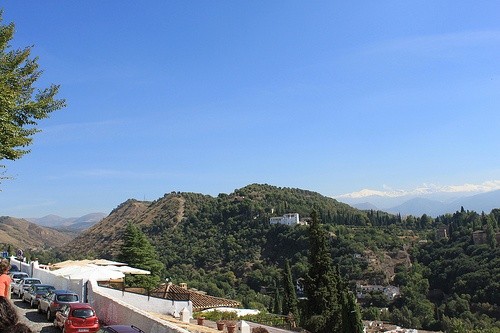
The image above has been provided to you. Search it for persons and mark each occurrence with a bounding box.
[0,258,10,299]
[0,295,32,333]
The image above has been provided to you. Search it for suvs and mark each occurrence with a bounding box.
[103,324,145,333]
[38,290,80,321]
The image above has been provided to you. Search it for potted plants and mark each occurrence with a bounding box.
[196,309,241,333]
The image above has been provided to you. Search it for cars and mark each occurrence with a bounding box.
[21,284,56,307]
[10,272,29,289]
[53,303,99,333]
[13,278,42,298]
